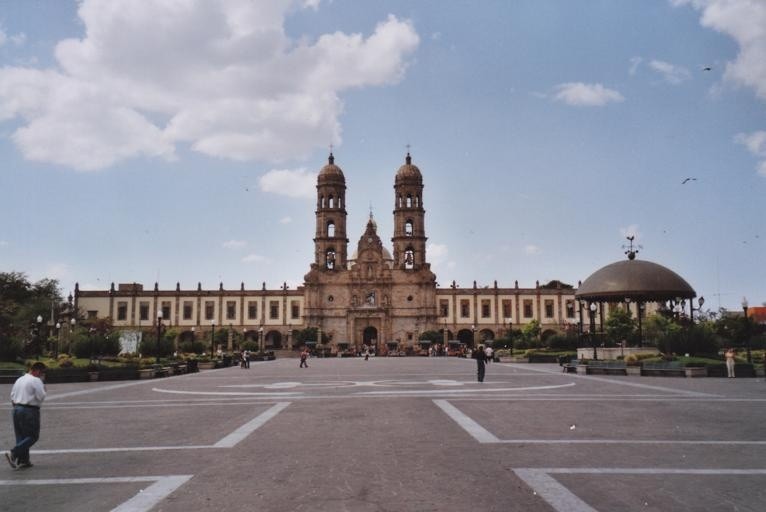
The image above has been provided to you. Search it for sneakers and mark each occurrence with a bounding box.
[18,459,33,467]
[5,451,17,468]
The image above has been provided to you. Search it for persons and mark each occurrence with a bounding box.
[724,347,736,378]
[4,362,50,469]
[475,345,489,381]
[172,340,497,370]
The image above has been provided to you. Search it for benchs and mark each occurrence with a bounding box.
[561,358,756,378]
[137,353,277,379]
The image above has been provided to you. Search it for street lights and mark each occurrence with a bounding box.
[70,318,76,332]
[681,300,687,314]
[509,318,513,355]
[210,320,215,359]
[538,324,542,337]
[259,326,264,352]
[191,327,195,348]
[471,325,475,350]
[55,322,60,360]
[625,296,631,313]
[638,302,646,345]
[590,303,598,361]
[567,298,583,334]
[691,297,705,320]
[156,310,163,363]
[741,297,753,364]
[243,328,247,341]
[35,314,42,360]
